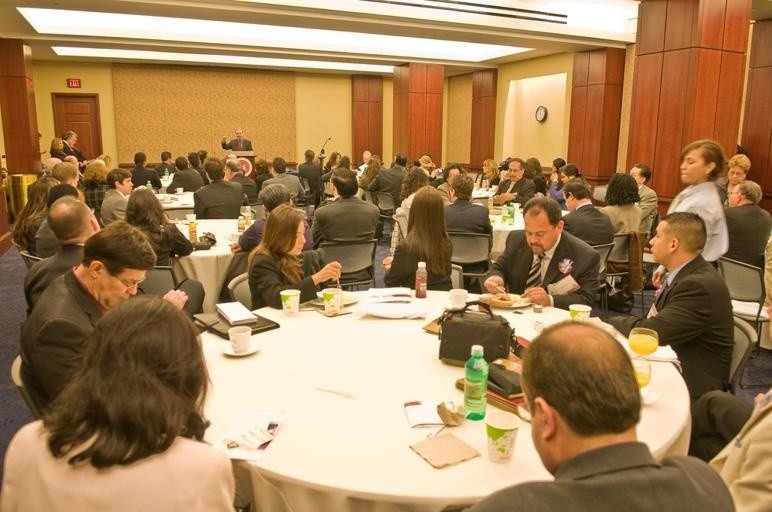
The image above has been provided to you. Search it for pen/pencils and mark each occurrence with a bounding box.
[426,425,446,438]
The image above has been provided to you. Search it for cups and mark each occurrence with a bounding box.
[176,188,184,193]
[280,289,300,318]
[228,326,251,351]
[485,410,522,465]
[450,288,468,310]
[322,288,343,316]
[569,304,592,322]
[628,328,658,356]
[186,213,196,222]
[628,358,652,388]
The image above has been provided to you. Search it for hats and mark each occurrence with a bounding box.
[419,156,436,168]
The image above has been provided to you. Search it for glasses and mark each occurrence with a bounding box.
[114,275,144,287]
[730,192,741,195]
[561,175,565,178]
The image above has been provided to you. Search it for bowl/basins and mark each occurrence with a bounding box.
[315,290,324,303]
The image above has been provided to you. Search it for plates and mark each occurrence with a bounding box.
[184,220,199,225]
[220,340,261,357]
[480,292,533,309]
[175,192,185,195]
[310,295,359,306]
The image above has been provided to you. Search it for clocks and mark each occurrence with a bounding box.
[536,107,547,122]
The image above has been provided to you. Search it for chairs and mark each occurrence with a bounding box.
[589,244,614,322]
[298,176,317,215]
[446,232,491,294]
[135,267,177,299]
[592,186,608,206]
[249,203,266,219]
[318,239,376,294]
[11,357,41,424]
[364,190,375,205]
[191,291,690,512]
[450,264,463,289]
[376,192,396,246]
[717,258,771,358]
[20,250,41,269]
[634,253,660,309]
[605,232,649,321]
[726,314,759,395]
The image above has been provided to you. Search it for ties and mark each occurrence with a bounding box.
[525,252,546,288]
[654,278,669,306]
[507,182,517,193]
[238,138,241,151]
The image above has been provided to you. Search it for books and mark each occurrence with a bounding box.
[456,359,526,415]
[216,302,259,327]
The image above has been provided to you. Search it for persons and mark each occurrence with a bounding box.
[475,159,500,188]
[254,159,273,197]
[45,158,61,176]
[221,127,254,151]
[462,320,734,512]
[653,140,728,289]
[484,198,598,312]
[63,131,88,174]
[551,164,590,210]
[723,181,772,274]
[716,154,751,203]
[548,158,566,190]
[83,160,109,209]
[562,181,613,247]
[299,150,324,209]
[0,295,235,512]
[691,238,772,511]
[125,189,193,266]
[598,173,641,273]
[321,157,350,182]
[50,138,67,162]
[419,155,437,181]
[354,151,372,173]
[323,152,341,173]
[446,175,494,273]
[13,177,62,255]
[390,169,428,257]
[437,163,465,207]
[230,183,314,255]
[368,155,409,242]
[359,155,383,190]
[64,156,85,191]
[35,184,78,257]
[101,169,134,224]
[166,157,206,193]
[20,221,189,402]
[198,150,207,168]
[154,151,175,177]
[248,204,342,311]
[188,152,210,186]
[491,158,534,207]
[383,186,453,291]
[262,157,307,207]
[631,165,659,245]
[194,159,244,220]
[99,155,112,171]
[498,157,511,185]
[131,152,162,190]
[609,212,734,402]
[224,158,258,203]
[311,168,380,281]
[23,198,206,325]
[525,158,546,196]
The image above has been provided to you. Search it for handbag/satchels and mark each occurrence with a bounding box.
[600,288,634,312]
[439,302,527,368]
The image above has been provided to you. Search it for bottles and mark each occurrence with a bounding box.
[147,181,151,190]
[508,205,515,224]
[416,262,427,298]
[238,216,244,232]
[165,168,169,177]
[464,344,488,421]
[189,220,196,242]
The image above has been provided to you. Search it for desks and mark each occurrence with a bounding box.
[231,151,257,182]
